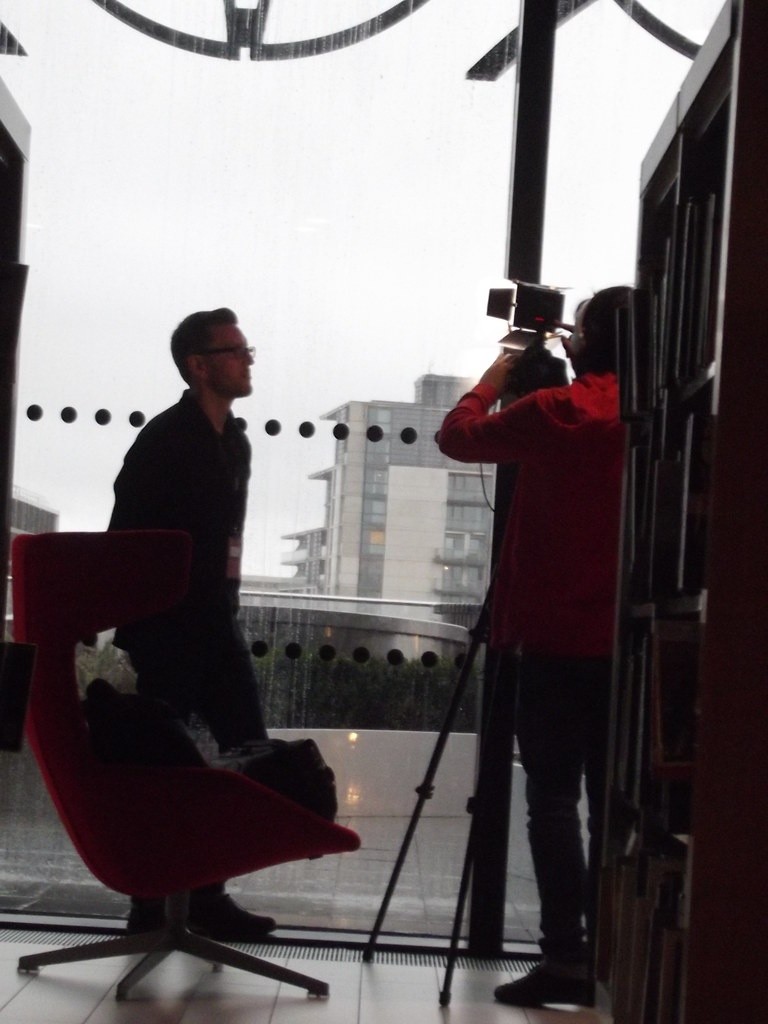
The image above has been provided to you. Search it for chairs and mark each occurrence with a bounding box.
[11,528,363,1001]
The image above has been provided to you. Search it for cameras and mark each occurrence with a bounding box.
[489,282,566,334]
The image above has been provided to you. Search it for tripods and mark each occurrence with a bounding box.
[362,563,517,1007]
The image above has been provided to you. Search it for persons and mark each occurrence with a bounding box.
[437,285,633,1005]
[105,307,277,936]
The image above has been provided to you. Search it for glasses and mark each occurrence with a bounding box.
[201,346,256,359]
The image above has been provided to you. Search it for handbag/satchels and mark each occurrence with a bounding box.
[205,740,337,823]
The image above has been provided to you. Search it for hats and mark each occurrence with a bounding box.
[577,286,634,359]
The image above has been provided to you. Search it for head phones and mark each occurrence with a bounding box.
[568,298,594,358]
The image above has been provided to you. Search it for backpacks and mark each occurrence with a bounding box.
[82,678,211,770]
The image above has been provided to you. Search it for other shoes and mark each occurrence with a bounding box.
[495,939,597,1008]
[128,893,277,940]
